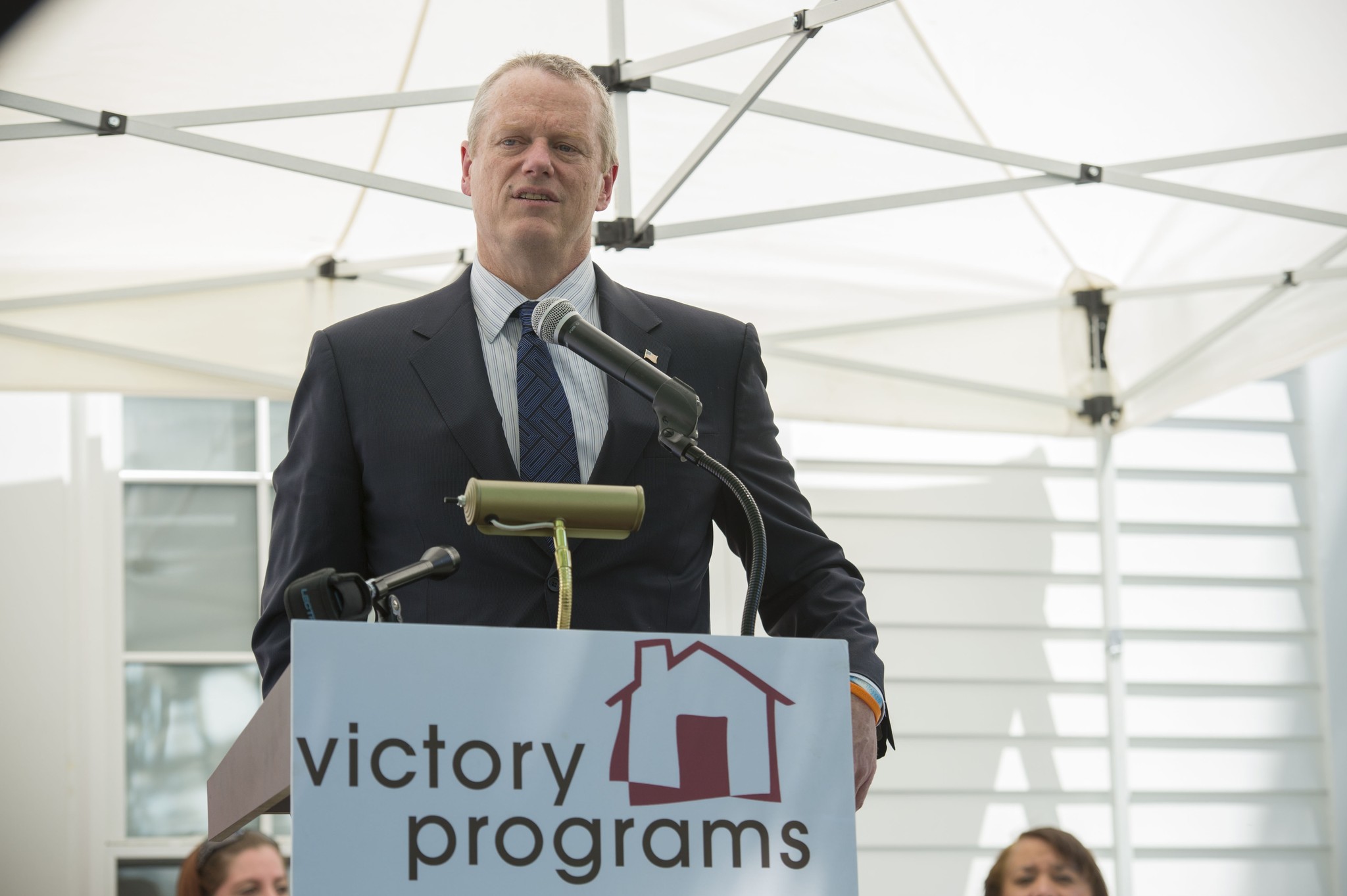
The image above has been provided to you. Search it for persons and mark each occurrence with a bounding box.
[176,826,288,896]
[250,55,896,895]
[984,828,1108,896]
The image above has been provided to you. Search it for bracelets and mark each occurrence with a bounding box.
[850,675,882,723]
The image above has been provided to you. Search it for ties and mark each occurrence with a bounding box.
[512,300,582,485]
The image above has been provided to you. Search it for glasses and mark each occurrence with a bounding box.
[196,828,246,875]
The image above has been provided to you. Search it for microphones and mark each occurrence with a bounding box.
[284,545,461,624]
[531,297,703,418]
